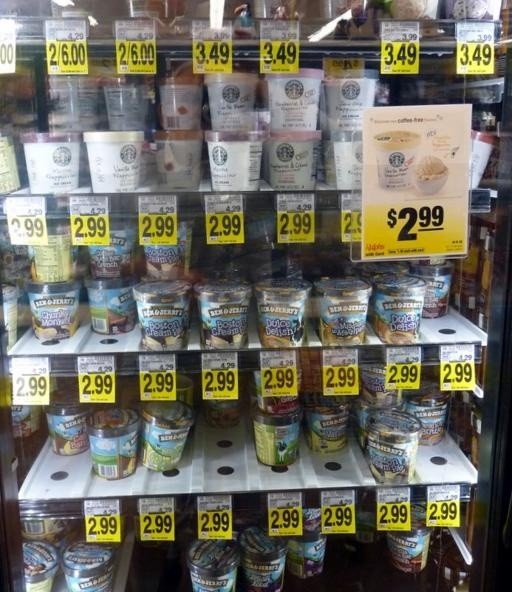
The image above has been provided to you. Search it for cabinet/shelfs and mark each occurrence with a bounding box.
[1,37,512,591]
